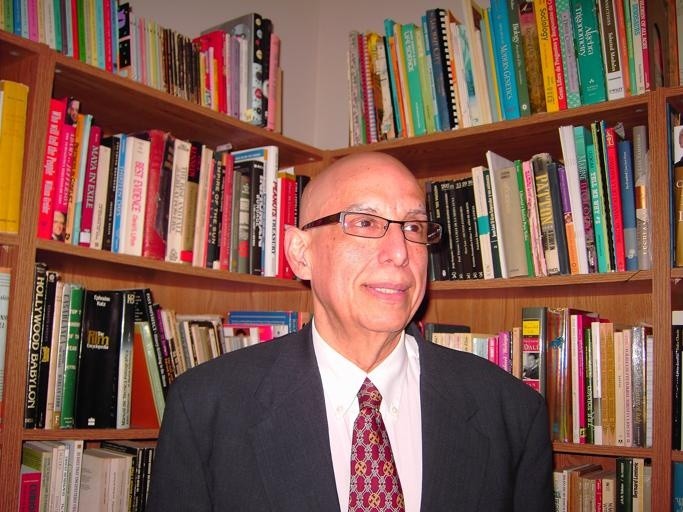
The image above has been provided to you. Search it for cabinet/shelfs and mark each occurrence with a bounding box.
[0,28,682,512]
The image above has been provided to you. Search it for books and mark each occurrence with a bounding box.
[1,1,282,135]
[672,461,683,512]
[417,307,652,448]
[666,102,683,269]
[18,440,156,512]
[672,311,683,453]
[0,81,312,279]
[1,263,311,430]
[427,121,650,281]
[346,1,664,147]
[553,456,652,512]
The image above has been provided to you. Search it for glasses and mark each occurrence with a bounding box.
[301,211,442,245]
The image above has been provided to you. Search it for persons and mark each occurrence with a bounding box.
[144,151,556,512]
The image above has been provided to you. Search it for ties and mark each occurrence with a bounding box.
[348,377,405,512]
